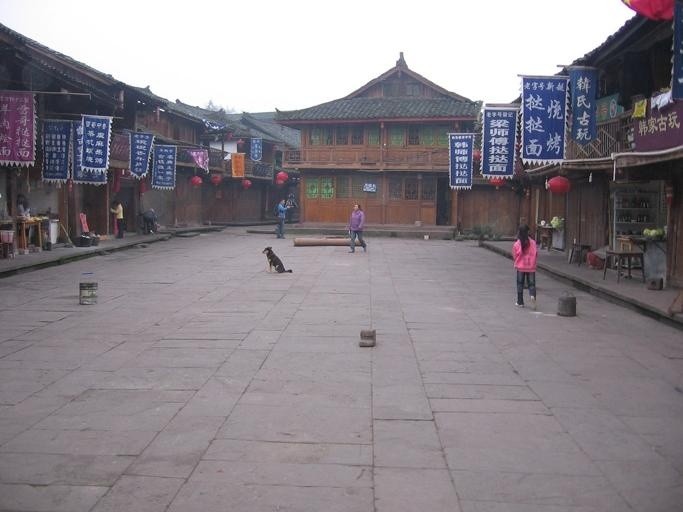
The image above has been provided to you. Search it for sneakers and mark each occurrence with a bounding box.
[364,246,366,252]
[514,302,524,309]
[530,295,538,310]
[348,251,354,253]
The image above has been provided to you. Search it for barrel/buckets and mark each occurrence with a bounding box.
[81,233,90,247]
[79,272,99,306]
[557,290,577,316]
[92,236,100,245]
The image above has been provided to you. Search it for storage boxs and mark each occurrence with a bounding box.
[0,229,15,243]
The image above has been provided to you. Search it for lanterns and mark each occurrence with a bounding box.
[490,177,506,190]
[548,175,571,194]
[474,151,481,160]
[621,0,675,21]
[276,171,288,184]
[211,175,222,185]
[192,176,202,184]
[241,180,252,190]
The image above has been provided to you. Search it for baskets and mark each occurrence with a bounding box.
[80,232,100,247]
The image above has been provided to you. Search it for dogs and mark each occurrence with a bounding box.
[262,246,293,274]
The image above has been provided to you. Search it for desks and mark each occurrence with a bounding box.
[541,228,553,251]
[603,251,645,283]
[18,219,43,250]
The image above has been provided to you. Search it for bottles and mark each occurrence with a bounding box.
[541,219,545,225]
[617,198,650,223]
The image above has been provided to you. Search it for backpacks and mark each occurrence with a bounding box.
[273,207,279,216]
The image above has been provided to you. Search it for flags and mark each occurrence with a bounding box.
[152,146,177,190]
[231,153,244,177]
[670,0,683,99]
[0,91,38,169]
[519,75,571,167]
[251,138,262,161]
[41,119,72,184]
[448,133,475,191]
[71,115,113,186]
[480,107,519,180]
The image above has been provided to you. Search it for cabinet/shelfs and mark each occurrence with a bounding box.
[616,179,656,237]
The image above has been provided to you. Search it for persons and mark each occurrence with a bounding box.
[513,225,537,308]
[110,200,124,239]
[16,194,30,216]
[276,199,290,239]
[139,208,157,233]
[347,204,367,253]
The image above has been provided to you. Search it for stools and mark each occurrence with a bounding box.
[569,243,591,266]
